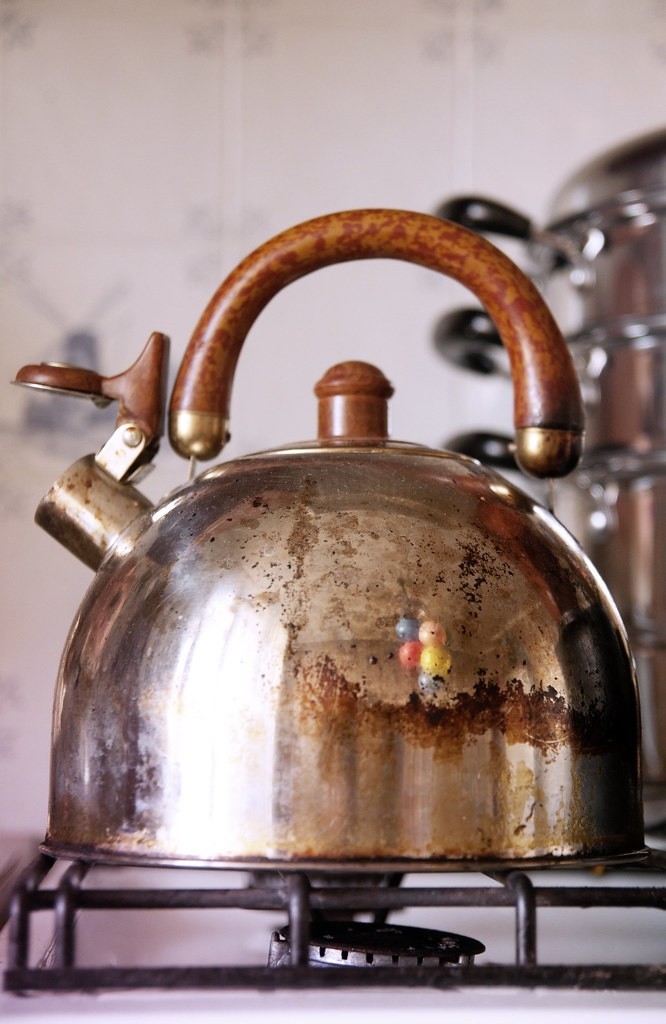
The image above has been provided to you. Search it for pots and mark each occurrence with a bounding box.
[433,123,666,845]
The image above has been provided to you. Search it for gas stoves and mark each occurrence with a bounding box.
[0,852,666,996]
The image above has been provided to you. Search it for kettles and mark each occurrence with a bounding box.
[10,208,654,874]
